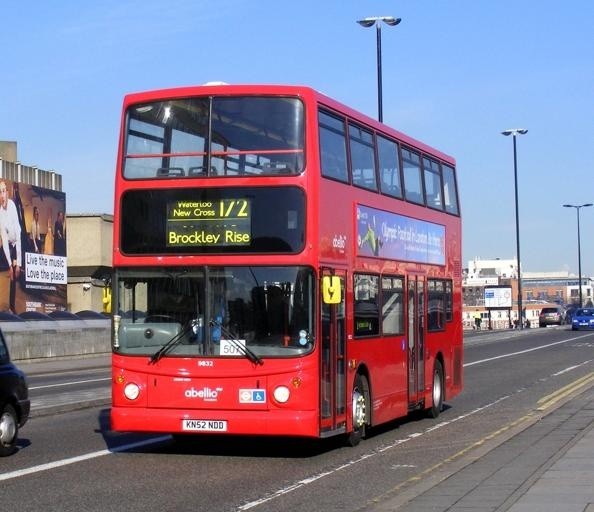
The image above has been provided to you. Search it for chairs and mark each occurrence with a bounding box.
[155,165,218,176]
[353,174,453,212]
[247,285,453,327]
[263,162,298,175]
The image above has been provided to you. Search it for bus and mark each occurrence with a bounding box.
[103,80,467,448]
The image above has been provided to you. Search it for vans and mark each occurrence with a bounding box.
[538,305,594,331]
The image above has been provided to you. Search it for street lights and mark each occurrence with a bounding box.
[561,204,594,308]
[355,14,404,124]
[499,128,531,331]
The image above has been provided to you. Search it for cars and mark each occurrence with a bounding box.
[1,323,39,455]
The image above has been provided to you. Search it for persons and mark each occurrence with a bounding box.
[0,182,65,279]
[473,309,481,329]
[360,214,383,256]
